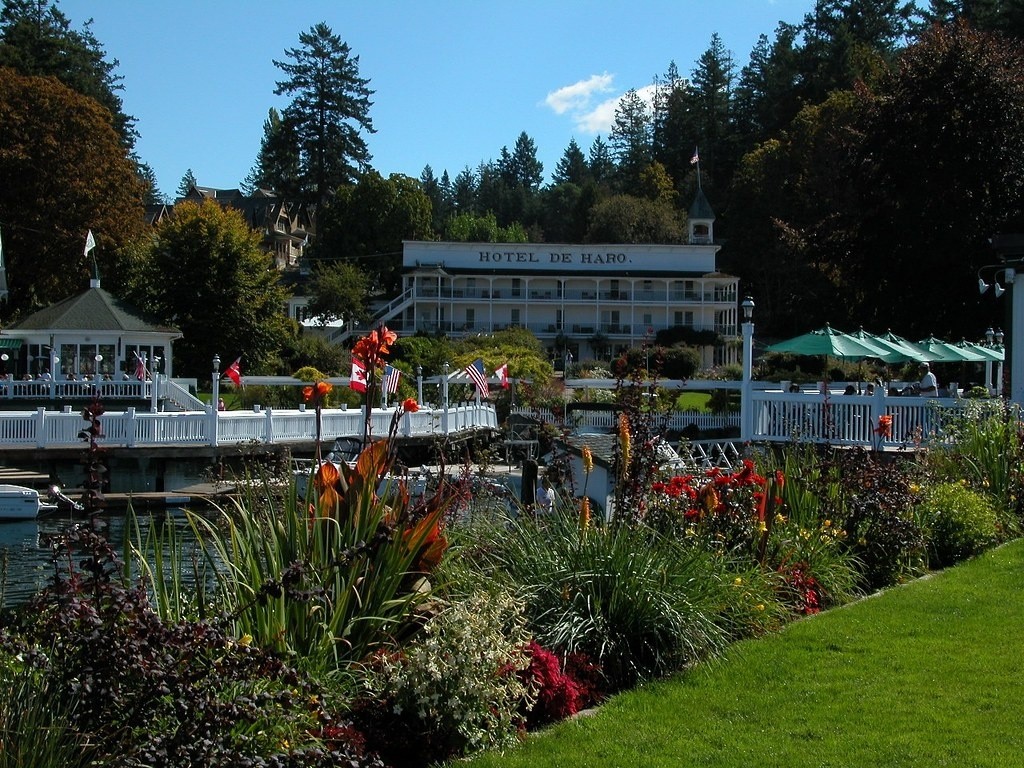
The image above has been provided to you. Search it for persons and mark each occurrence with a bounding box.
[0,368,129,395]
[780,362,976,441]
[218,397,225,410]
[536,477,556,515]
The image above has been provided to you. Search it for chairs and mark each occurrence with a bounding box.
[494,324,499,331]
[691,293,711,301]
[764,401,780,435]
[532,291,537,299]
[549,325,555,333]
[608,325,631,334]
[582,291,628,300]
[573,325,580,333]
[482,290,500,298]
[545,292,551,299]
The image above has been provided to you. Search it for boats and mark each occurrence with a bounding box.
[285,435,508,506]
[0,484,86,521]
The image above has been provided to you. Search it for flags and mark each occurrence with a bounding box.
[348,357,367,393]
[495,363,508,390]
[225,357,242,388]
[466,357,490,399]
[134,359,143,380]
[382,363,400,394]
[83,230,95,259]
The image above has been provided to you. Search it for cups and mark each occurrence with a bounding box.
[913,383,919,390]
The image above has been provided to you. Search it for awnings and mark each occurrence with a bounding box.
[0,339,25,348]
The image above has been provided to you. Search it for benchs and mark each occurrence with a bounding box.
[581,328,593,333]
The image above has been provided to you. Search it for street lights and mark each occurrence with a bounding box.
[442,361,449,411]
[210,354,222,414]
[985,327,994,389]
[150,355,159,413]
[416,365,423,407]
[564,349,576,426]
[995,327,1005,397]
[740,296,756,440]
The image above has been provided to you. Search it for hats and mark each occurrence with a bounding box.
[918,362,929,368]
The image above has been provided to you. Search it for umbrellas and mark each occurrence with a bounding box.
[878,328,944,398]
[913,332,987,373]
[846,324,927,397]
[952,337,1005,392]
[763,320,894,396]
